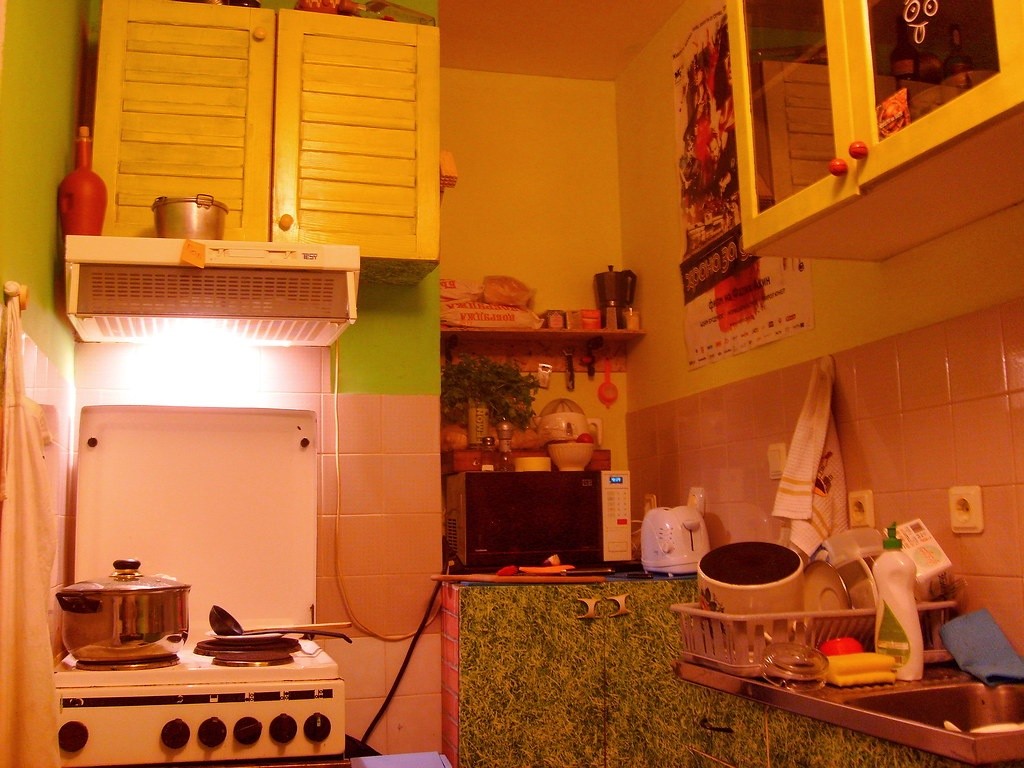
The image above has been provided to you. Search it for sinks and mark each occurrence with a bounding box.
[840,681,1024,738]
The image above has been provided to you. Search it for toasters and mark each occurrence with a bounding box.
[639,505,710,578]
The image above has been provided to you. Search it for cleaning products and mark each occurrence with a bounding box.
[871,521,924,681]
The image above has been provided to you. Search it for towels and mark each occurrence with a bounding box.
[770,361,849,558]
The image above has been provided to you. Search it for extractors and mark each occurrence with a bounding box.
[64,237,361,348]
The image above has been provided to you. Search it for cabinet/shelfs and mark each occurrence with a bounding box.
[90,0,439,283]
[440,581,969,768]
[724,0,1024,261]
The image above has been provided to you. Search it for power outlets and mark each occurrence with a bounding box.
[644,494,656,511]
[948,486,984,534]
[848,490,874,529]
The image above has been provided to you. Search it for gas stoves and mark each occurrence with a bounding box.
[53,636,346,768]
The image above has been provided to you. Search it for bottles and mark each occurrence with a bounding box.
[338,2,436,27]
[889,17,920,102]
[947,23,973,78]
[872,521,922,681]
[478,420,516,472]
[61,124,108,235]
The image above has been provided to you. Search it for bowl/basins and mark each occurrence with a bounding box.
[549,443,596,473]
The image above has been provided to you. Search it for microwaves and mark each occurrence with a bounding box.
[443,470,632,568]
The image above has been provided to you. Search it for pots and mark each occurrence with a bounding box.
[152,194,231,241]
[697,542,807,644]
[55,559,192,661]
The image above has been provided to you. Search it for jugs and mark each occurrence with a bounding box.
[532,399,602,447]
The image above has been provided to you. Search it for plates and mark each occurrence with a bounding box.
[803,561,851,614]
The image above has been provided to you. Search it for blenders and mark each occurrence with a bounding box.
[594,265,637,331]
